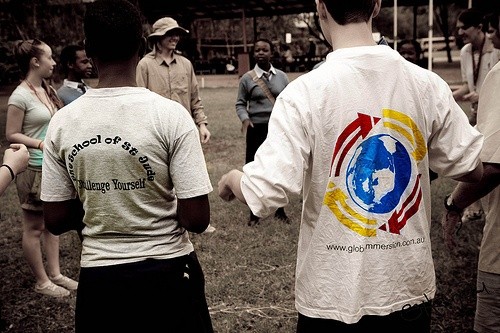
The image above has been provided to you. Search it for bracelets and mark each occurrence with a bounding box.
[37,140,43,150]
[0,164,15,180]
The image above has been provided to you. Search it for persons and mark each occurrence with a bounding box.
[55,44,94,106]
[236,39,292,224]
[487,8,500,48]
[215,0,484,333]
[136,17,216,233]
[396,38,422,65]
[39,0,213,333]
[451,7,500,223]
[5,38,79,297]
[441,62,500,333]
[0,143,30,195]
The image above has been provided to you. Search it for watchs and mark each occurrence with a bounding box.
[444,192,464,214]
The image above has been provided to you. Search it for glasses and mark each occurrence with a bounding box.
[32,37,41,45]
[81,58,93,64]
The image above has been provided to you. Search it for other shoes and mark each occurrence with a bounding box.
[462,209,484,224]
[275,207,290,224]
[247,210,260,226]
[49,275,78,289]
[205,224,215,233]
[34,280,69,297]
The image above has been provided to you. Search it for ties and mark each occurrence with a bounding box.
[78,85,86,94]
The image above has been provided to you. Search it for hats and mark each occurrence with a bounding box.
[146,17,189,40]
